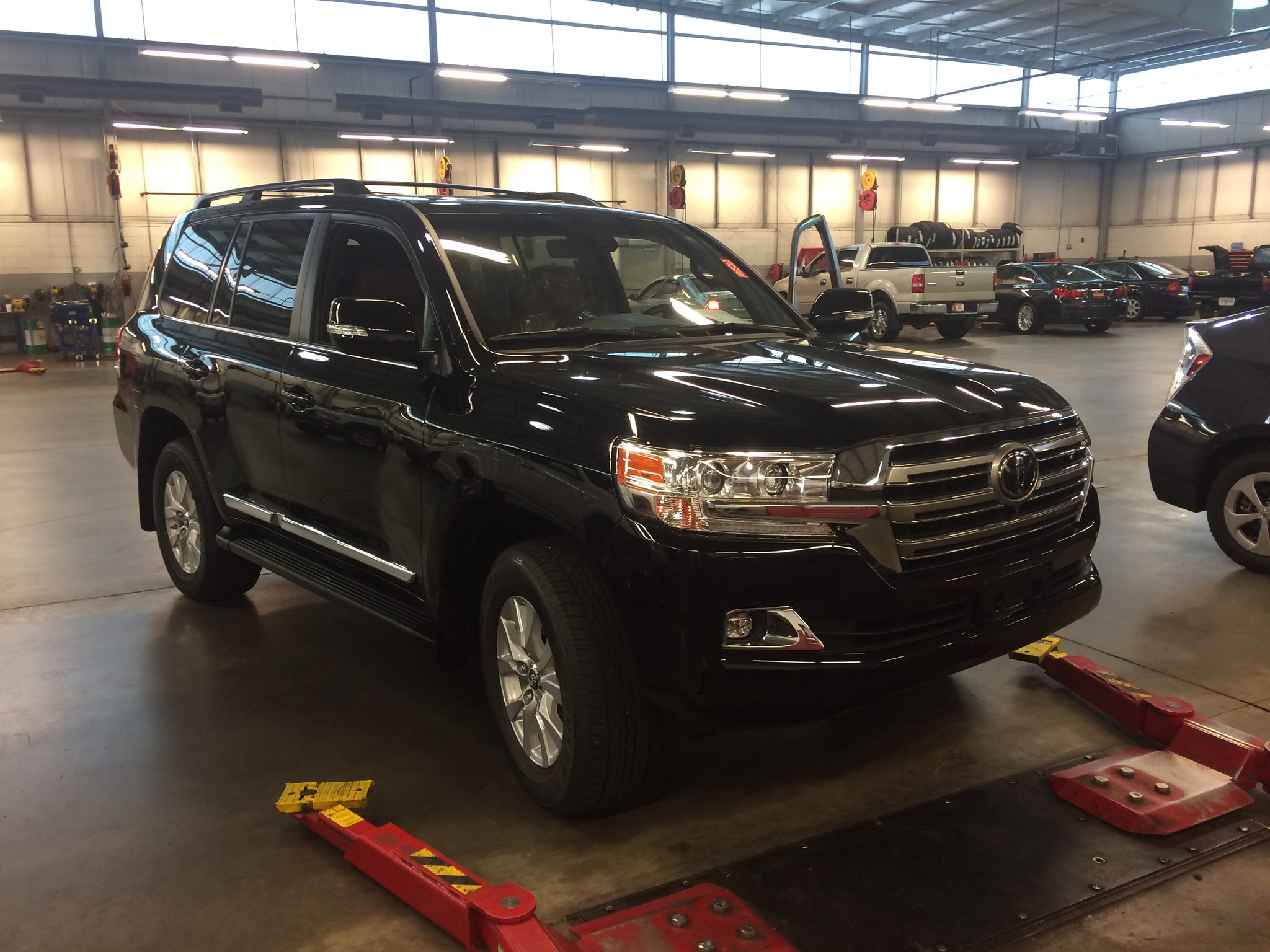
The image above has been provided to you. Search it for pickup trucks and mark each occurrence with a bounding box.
[769,240,995,343]
[1191,242,1270,320]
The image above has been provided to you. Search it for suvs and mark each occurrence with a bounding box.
[107,173,1106,812]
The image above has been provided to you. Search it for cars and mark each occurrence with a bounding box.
[1142,304,1270,571]
[990,258,1115,333]
[1085,255,1201,321]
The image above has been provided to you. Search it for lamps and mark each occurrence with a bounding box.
[429,0,582,87]
[1016,2,1270,131]
[659,1,792,102]
[136,1,321,69]
[857,14,964,112]
